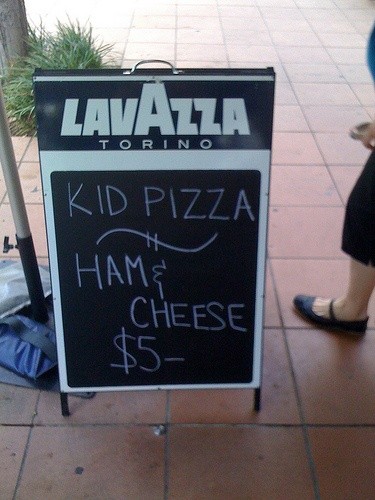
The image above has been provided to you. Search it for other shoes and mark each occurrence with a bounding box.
[292,295,369,335]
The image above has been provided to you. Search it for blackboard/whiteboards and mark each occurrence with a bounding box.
[30,60,276,394]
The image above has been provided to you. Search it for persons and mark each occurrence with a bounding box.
[293,19,375,335]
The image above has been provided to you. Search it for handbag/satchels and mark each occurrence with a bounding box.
[1,315,57,381]
